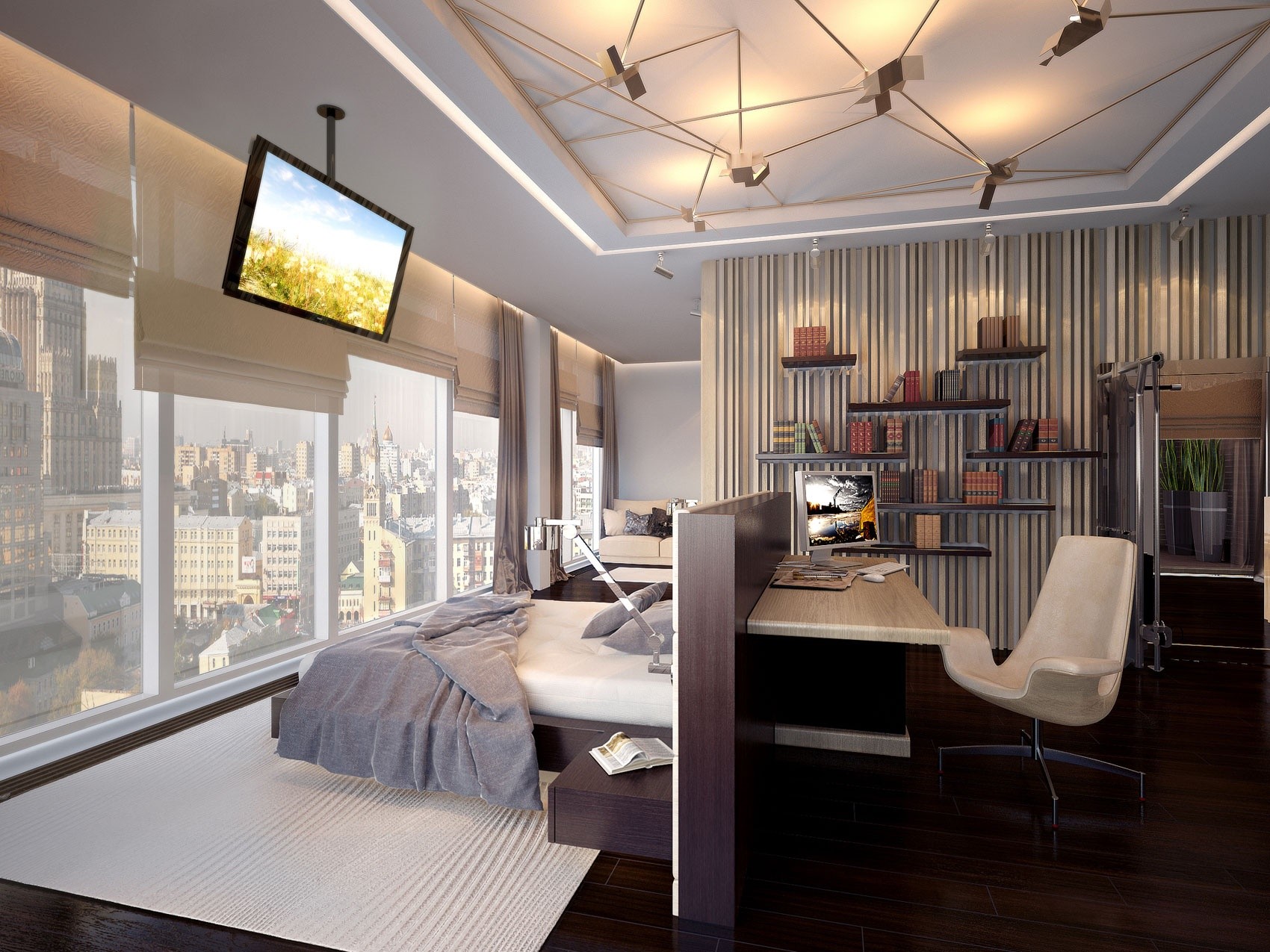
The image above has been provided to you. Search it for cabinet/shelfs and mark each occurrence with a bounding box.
[754,345,1104,557]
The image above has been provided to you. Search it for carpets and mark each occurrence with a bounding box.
[592,567,672,583]
[0,687,600,952]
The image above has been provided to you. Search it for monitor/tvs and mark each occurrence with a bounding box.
[220,133,415,344]
[795,470,881,568]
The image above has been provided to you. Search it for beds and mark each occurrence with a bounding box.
[271,491,790,929]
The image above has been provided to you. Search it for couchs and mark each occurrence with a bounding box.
[599,499,702,565]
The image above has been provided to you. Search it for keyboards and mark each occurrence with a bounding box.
[855,562,910,575]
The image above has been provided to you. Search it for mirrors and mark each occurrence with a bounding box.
[1159,437,1261,575]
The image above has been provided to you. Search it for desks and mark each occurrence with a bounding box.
[748,556,951,758]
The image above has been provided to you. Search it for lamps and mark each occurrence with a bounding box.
[1171,204,1196,243]
[522,516,672,675]
[981,223,995,256]
[810,239,822,268]
[653,252,674,280]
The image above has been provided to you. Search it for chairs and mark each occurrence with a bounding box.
[937,536,1147,828]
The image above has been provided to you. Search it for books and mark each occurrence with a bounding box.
[773,315,1060,549]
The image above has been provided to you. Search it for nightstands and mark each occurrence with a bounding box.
[548,732,672,860]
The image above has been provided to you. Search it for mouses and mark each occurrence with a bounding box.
[863,574,885,583]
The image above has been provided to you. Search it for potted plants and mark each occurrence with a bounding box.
[1160,439,1227,562]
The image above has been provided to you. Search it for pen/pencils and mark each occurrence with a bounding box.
[804,575,841,579]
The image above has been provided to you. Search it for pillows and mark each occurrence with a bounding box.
[581,580,669,638]
[601,600,673,655]
[603,508,672,538]
